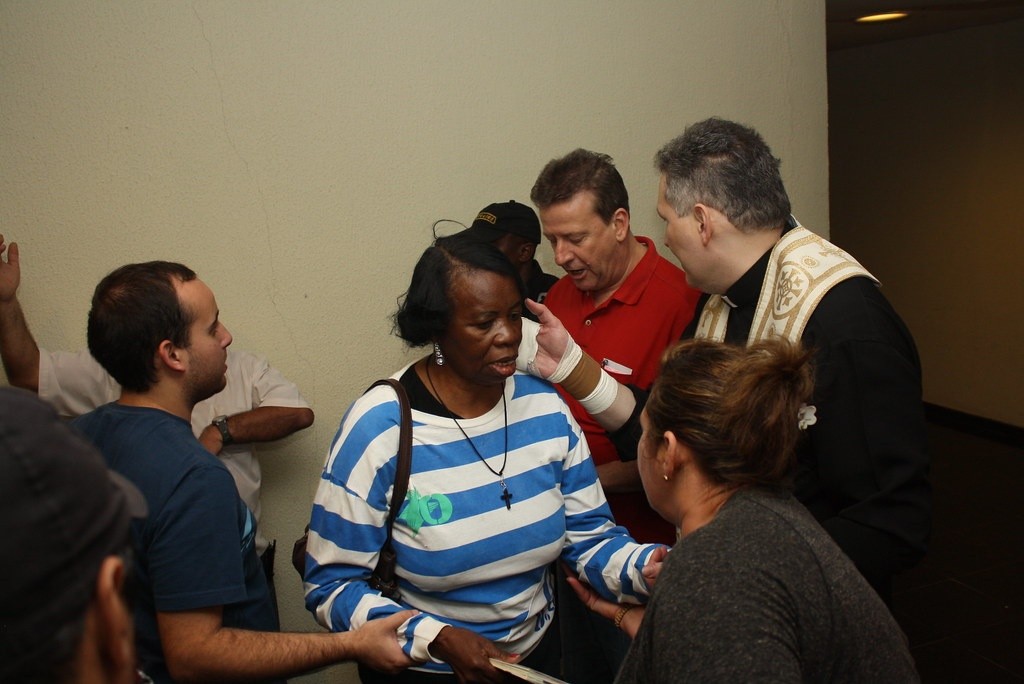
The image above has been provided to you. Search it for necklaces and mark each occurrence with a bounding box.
[427,354,513,510]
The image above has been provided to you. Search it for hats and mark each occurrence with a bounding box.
[467,200,541,245]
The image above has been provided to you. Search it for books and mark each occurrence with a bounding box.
[488,658,567,684]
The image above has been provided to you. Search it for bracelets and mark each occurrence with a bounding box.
[615,604,635,632]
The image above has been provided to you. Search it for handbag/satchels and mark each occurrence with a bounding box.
[292,381,413,613]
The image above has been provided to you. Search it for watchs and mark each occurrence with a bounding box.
[212,415,232,445]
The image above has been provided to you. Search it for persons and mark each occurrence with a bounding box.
[0,234,315,544]
[464,201,561,321]
[305,237,674,684]
[0,385,149,684]
[518,117,934,612]
[63,262,425,684]
[569,339,919,683]
[530,148,704,684]
